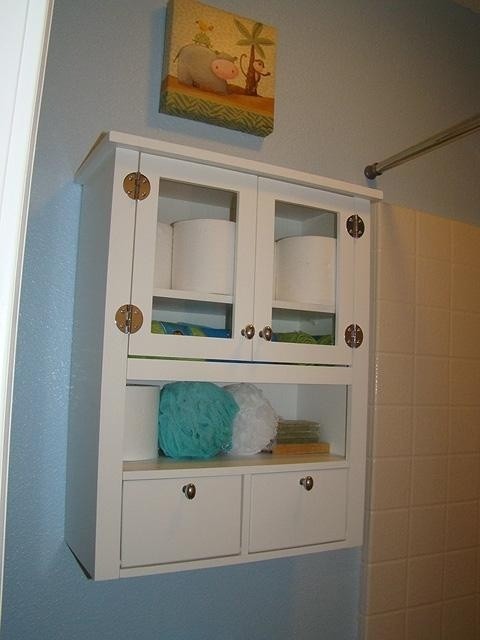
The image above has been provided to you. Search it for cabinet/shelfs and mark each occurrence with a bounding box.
[64,131,384,582]
